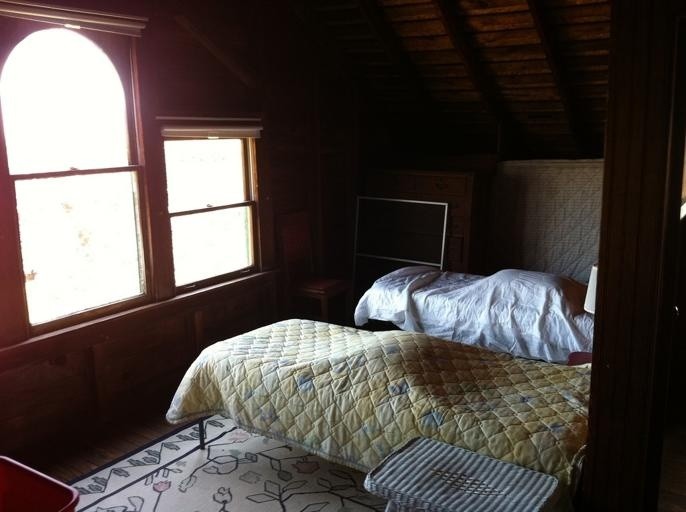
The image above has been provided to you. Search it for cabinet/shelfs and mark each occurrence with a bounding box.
[364,170,488,273]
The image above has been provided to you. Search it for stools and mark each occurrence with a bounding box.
[364,436,560,512]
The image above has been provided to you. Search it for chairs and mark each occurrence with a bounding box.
[275,210,351,323]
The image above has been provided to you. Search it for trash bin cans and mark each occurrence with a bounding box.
[0,455,80,512]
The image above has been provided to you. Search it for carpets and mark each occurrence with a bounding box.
[66,415,388,511]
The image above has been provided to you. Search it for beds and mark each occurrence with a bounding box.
[165,320,591,511]
[353,261,594,365]
